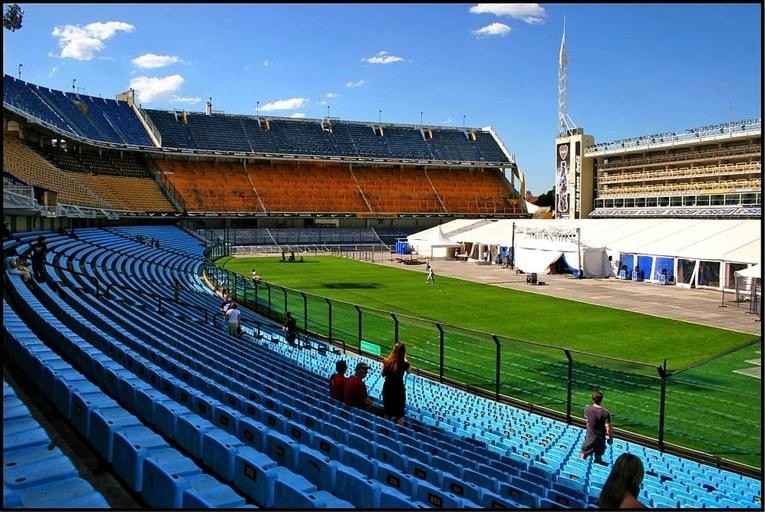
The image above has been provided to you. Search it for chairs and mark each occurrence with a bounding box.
[0,72,521,216]
[0,222,763,508]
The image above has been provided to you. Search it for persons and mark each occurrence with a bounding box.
[597,454,650,509]
[3,221,13,241]
[211,250,306,347]
[580,389,614,467]
[378,341,410,428]
[330,362,353,398]
[130,234,161,250]
[425,266,436,285]
[346,361,373,412]
[31,235,48,284]
[4,247,32,287]
[452,246,492,264]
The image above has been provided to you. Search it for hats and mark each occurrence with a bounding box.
[356,363,371,369]
